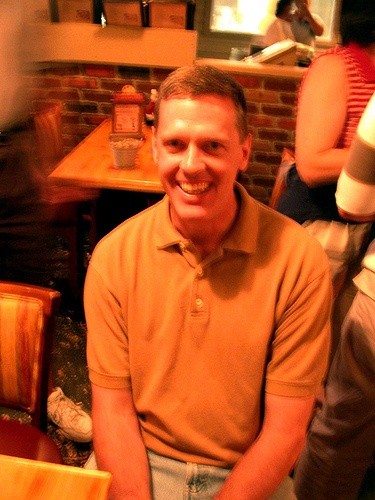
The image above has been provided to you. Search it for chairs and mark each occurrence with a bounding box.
[24,105,103,307]
[0,280,63,464]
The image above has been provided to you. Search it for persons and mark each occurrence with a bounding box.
[1,0,94,443]
[262,0,303,64]
[274,0,375,304]
[292,89,375,500]
[288,0,324,66]
[83,64,334,500]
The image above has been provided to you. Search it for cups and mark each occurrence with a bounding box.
[109,137,143,169]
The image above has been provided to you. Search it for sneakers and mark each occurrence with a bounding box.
[46,386,93,443]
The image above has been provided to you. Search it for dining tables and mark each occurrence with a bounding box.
[49,116,168,213]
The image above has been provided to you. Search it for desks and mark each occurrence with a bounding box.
[0,454,114,500]
